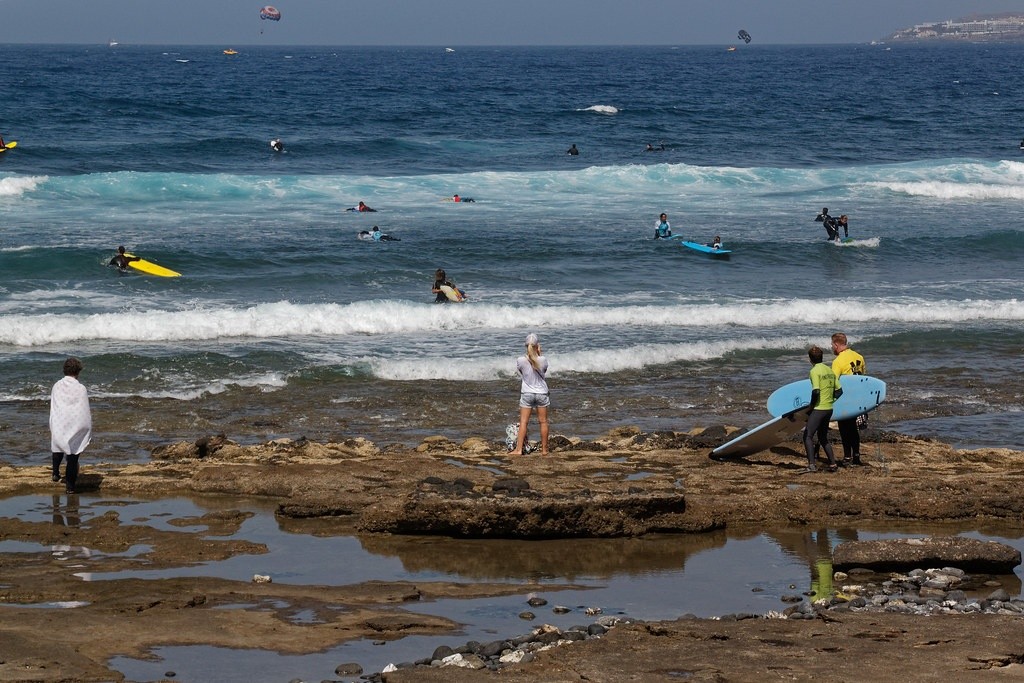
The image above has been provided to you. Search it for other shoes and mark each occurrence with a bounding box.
[804,465,817,472]
[850,459,862,467]
[838,459,851,468]
[66,490,74,494]
[825,465,837,471]
[52,475,61,482]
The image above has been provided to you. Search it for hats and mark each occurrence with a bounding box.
[524,333,538,346]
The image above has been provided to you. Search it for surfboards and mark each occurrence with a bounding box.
[708,405,811,459]
[766,375,886,421]
[682,241,732,255]
[439,284,468,304]
[122,253,183,279]
[839,239,856,243]
[1,141,18,153]
[271,141,288,154]
[659,235,684,241]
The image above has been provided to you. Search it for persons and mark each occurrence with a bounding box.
[643,140,666,152]
[799,524,842,606]
[273,139,284,153]
[815,206,832,222]
[48,494,94,607]
[654,213,672,240]
[432,268,455,302]
[508,333,551,456]
[49,356,95,494]
[700,236,723,250]
[823,215,848,240]
[454,194,475,202]
[831,333,867,468]
[109,246,140,271]
[361,226,401,243]
[347,202,377,213]
[524,578,543,603]
[831,526,862,605]
[567,143,579,155]
[803,347,843,472]
